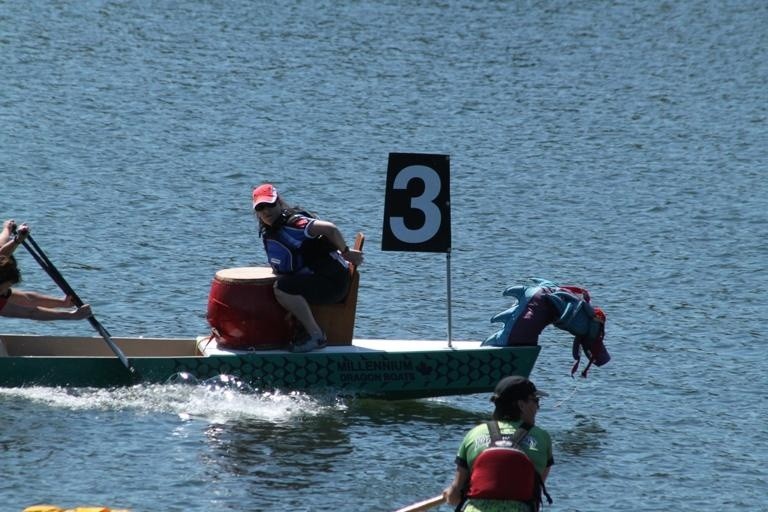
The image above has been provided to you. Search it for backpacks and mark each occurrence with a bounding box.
[459,420,538,501]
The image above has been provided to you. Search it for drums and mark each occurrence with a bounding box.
[208,265,313,352]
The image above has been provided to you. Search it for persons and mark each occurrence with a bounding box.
[0,258,93,323]
[250,182,366,354]
[0,219,32,257]
[437,374,557,512]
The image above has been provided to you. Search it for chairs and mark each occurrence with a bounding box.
[294,232,366,346]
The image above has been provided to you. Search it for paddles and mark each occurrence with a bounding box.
[21,223,150,384]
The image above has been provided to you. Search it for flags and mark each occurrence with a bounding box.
[381,151,452,255]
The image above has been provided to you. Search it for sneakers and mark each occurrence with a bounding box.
[290,327,329,353]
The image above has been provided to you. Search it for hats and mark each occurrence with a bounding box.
[252,183,278,210]
[493,376,537,401]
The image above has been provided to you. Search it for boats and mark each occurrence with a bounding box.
[0,330,543,402]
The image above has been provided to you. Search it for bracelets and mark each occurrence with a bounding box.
[341,246,350,255]
[14,236,22,244]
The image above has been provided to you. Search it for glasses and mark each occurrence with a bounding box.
[528,398,540,404]
